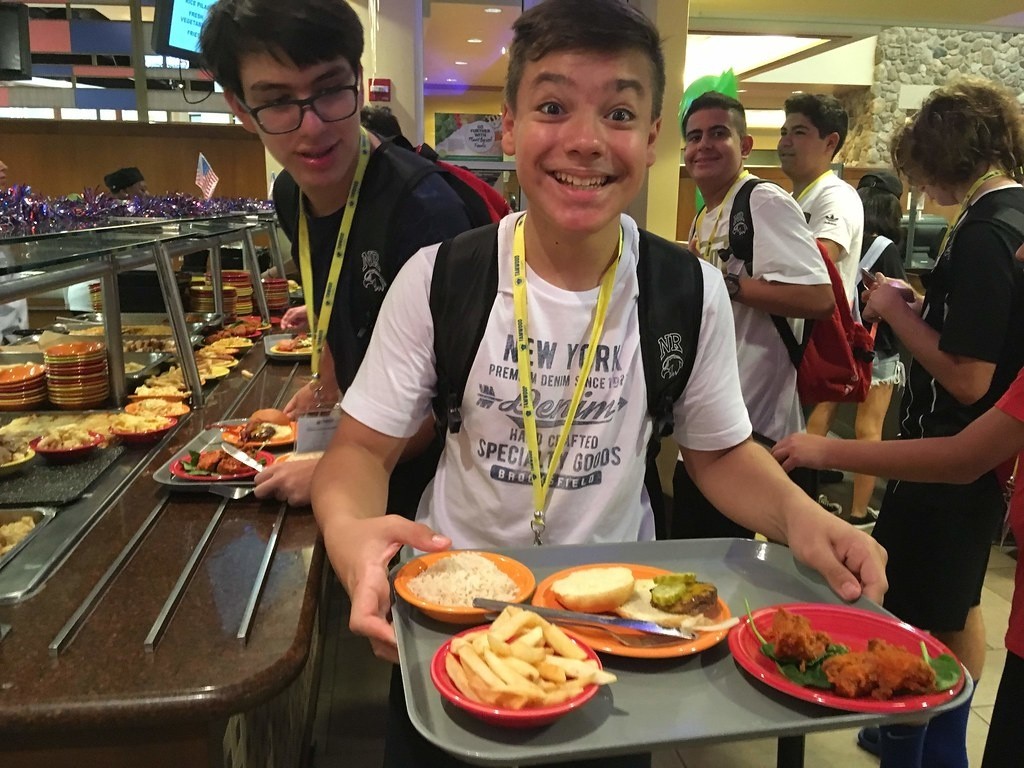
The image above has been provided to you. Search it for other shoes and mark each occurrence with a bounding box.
[818,467,844,484]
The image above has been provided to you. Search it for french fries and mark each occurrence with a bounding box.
[144,364,212,387]
[444,606,617,708]
[242,370,253,377]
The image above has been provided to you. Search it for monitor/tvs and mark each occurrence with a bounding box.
[151,0,221,64]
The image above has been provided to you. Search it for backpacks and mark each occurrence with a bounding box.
[690,178,874,406]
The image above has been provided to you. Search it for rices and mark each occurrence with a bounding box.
[406,552,520,606]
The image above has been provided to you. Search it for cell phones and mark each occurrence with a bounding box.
[861,267,878,282]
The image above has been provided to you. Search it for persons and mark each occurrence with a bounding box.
[199,0,515,570]
[673,92,835,544]
[778,92,864,316]
[310,0,888,768]
[807,172,925,527]
[770,78,1024,768]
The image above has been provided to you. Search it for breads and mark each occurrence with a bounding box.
[551,567,704,626]
[249,408,291,426]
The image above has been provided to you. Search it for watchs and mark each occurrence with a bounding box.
[724,273,740,300]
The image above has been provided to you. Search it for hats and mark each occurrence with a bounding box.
[856,171,902,200]
[103,168,144,193]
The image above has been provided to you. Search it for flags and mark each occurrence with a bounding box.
[196,152,219,202]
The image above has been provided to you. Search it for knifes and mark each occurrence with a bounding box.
[208,485,256,499]
[222,443,264,473]
[473,598,694,639]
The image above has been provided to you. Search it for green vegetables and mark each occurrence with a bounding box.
[179,449,265,476]
[230,318,268,327]
[744,601,961,691]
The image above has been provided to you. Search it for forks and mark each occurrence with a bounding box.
[484,611,690,646]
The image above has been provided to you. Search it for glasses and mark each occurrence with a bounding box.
[231,68,360,134]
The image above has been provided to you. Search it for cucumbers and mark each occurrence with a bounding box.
[652,572,696,585]
[651,585,687,605]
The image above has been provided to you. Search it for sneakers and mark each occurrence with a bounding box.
[818,494,843,514]
[848,504,881,529]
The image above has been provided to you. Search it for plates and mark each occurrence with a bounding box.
[532,564,731,658]
[727,603,966,713]
[0,268,311,481]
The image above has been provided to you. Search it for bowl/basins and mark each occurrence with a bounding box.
[431,625,601,727]
[394,551,535,623]
